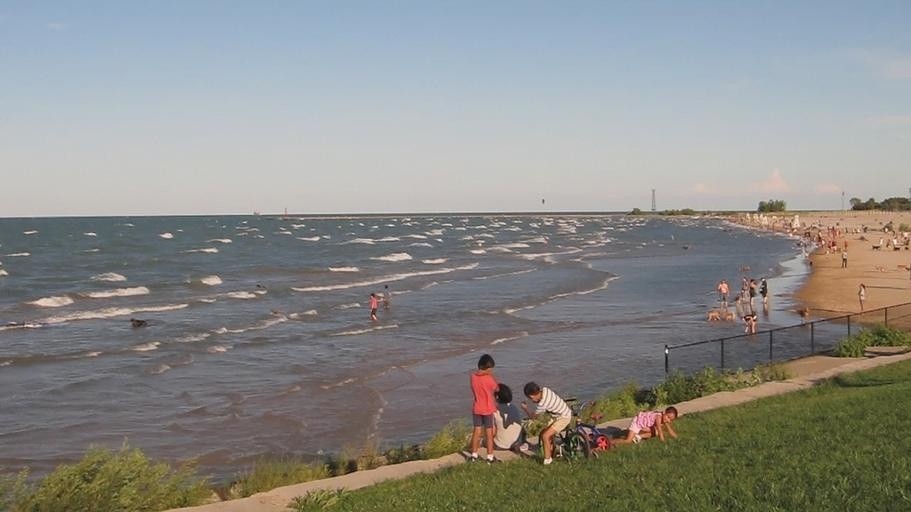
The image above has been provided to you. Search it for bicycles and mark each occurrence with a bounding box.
[522,397,611,464]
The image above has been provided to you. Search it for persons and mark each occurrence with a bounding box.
[520,381,572,465]
[479,383,530,451]
[382,285,392,310]
[716,212,911,333]
[609,406,678,445]
[469,354,500,465]
[369,292,378,320]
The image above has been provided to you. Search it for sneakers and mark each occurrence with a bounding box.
[631,433,643,445]
[466,442,554,468]
